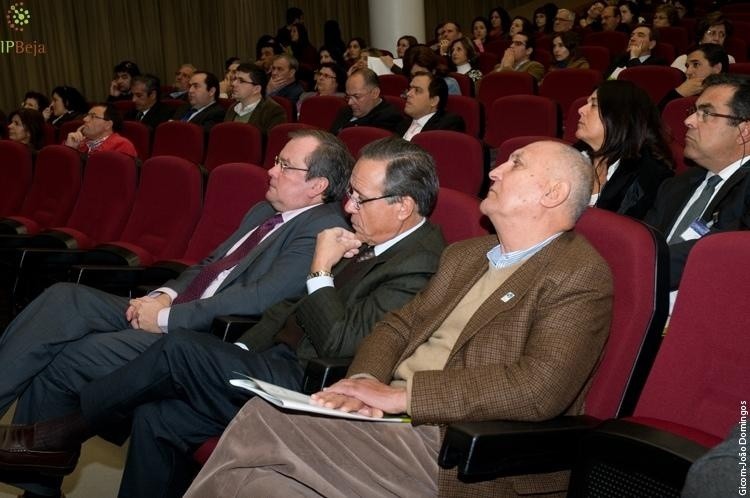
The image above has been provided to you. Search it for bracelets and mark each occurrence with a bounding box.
[305,271,335,280]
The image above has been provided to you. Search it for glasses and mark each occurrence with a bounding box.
[686,106,743,123]
[345,90,369,101]
[347,191,392,210]
[232,76,254,85]
[317,71,335,79]
[84,112,104,121]
[274,156,309,172]
[509,39,527,47]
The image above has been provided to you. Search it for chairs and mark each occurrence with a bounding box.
[0,0,428,497]
[454,229,750,497]
[429,1,750,250]
[428,201,669,498]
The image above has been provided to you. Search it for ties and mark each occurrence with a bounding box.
[172,213,283,304]
[668,174,723,243]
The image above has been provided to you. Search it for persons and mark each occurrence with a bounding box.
[0,138,448,498]
[178,140,616,496]
[2,1,750,412]
[0,129,354,498]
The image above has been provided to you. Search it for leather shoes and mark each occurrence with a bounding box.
[1,425,80,474]
